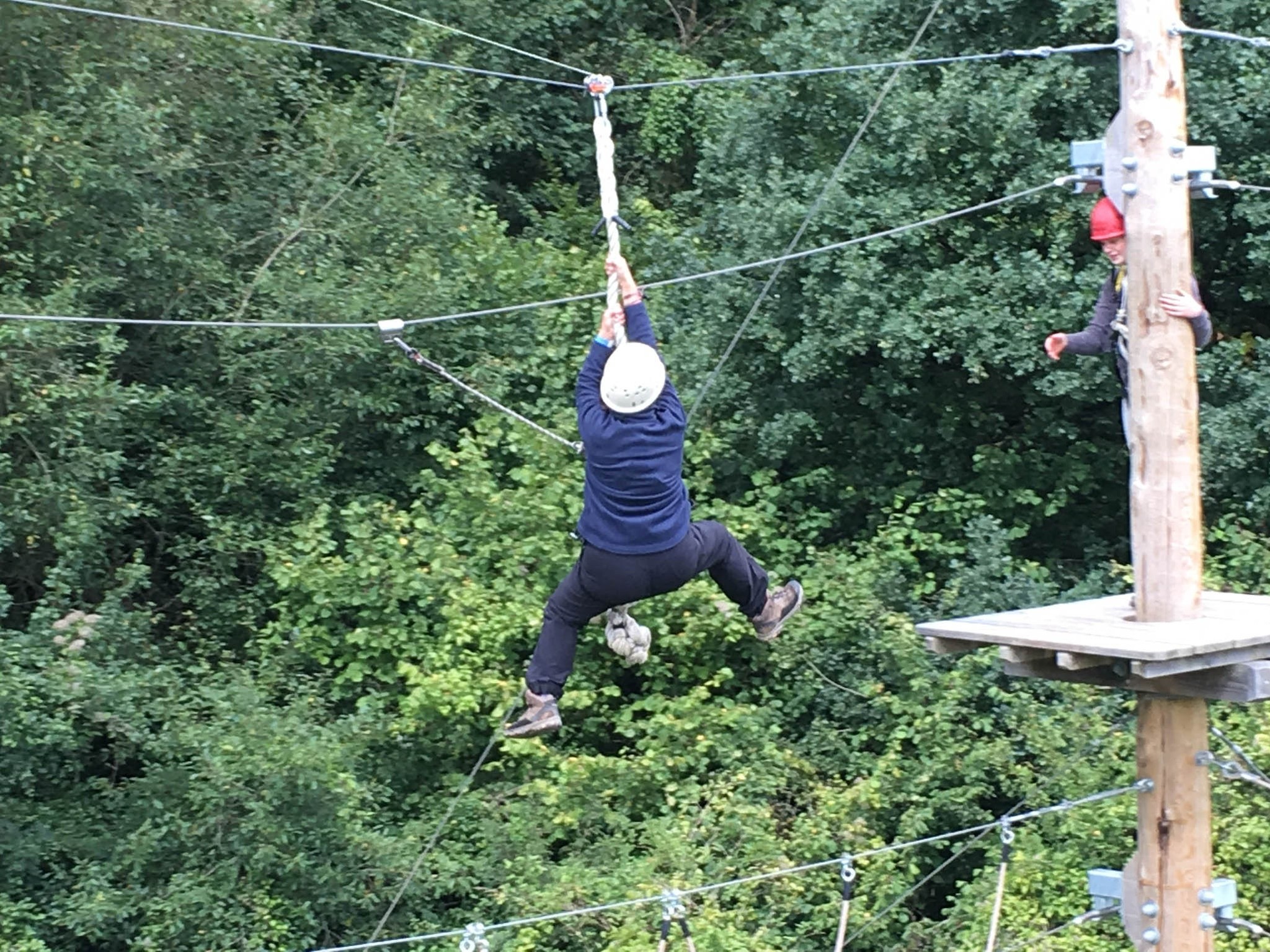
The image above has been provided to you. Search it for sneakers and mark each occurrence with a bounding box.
[503,689,562,738]
[747,580,803,639]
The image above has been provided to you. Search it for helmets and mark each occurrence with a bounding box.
[1089,198,1125,240]
[600,342,666,414]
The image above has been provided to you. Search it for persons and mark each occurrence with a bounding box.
[1045,197,1212,453]
[502,251,804,738]
